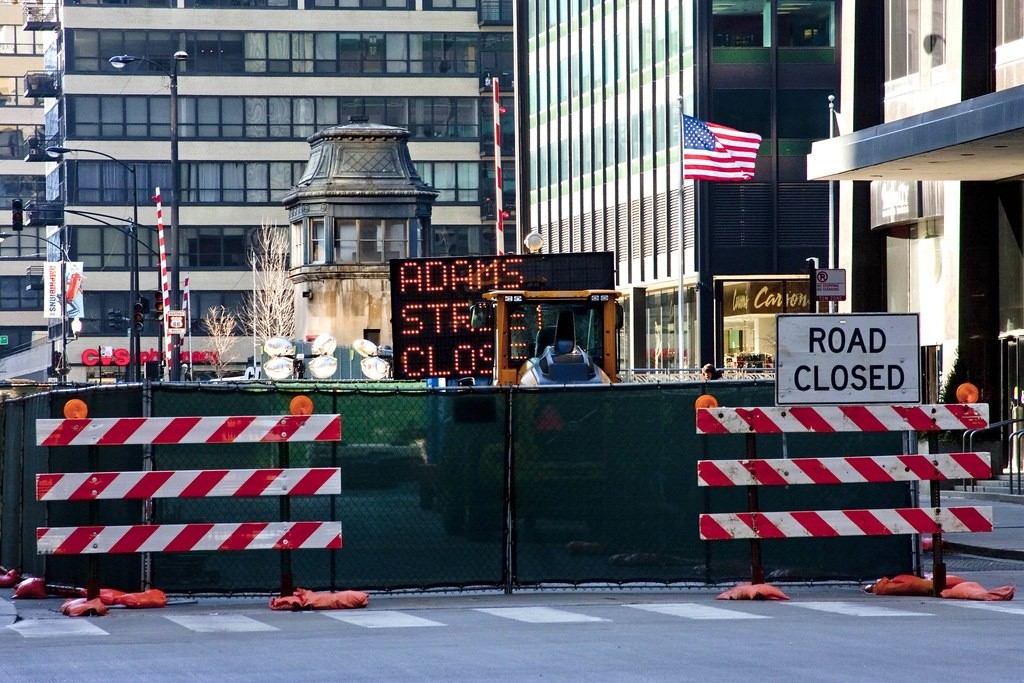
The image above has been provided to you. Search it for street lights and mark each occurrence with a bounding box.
[43,146,139,382]
[0,232,67,386]
[109,51,189,380]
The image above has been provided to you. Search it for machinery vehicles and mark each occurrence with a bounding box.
[472,290,624,388]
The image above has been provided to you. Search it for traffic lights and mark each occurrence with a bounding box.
[54,351,61,377]
[156,290,164,322]
[134,302,144,331]
[11,199,23,231]
[116,311,123,328]
[110,309,116,326]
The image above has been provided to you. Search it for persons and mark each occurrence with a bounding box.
[701,364,723,380]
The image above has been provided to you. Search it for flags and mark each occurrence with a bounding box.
[683,114,762,183]
